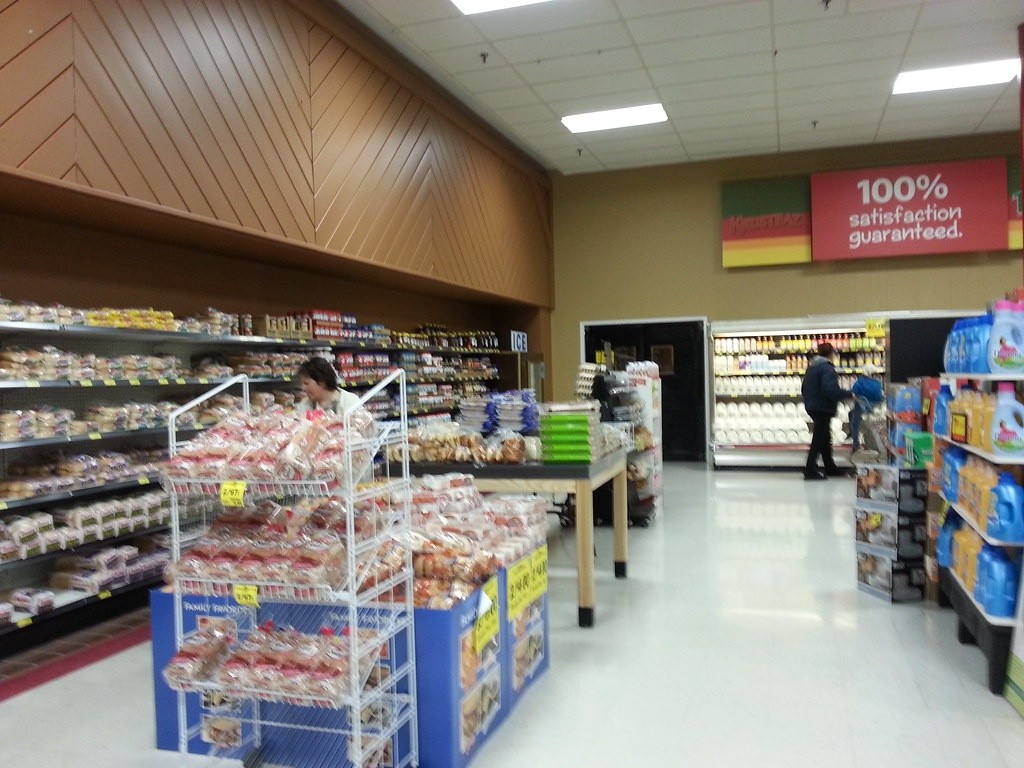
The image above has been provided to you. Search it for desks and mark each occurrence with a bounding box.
[382,446,631,628]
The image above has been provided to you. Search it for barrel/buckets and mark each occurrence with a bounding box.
[936,297,1024,617]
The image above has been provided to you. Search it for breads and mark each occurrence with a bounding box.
[0,391,307,440]
[0,347,309,380]
[164,405,545,704]
[1,303,175,333]
[0,448,167,501]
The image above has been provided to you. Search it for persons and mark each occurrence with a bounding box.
[998,420,1019,444]
[998,339,1018,362]
[800,342,855,478]
[843,363,886,453]
[290,354,379,442]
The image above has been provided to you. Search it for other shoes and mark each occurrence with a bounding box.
[803,472,827,480]
[826,468,844,477]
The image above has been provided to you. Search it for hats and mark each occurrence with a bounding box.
[818,343,833,357]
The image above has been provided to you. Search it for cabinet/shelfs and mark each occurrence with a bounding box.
[167,366,420,768]
[932,374,1024,696]
[708,329,887,469]
[0,323,500,701]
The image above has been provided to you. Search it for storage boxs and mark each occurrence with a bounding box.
[651,345,675,375]
[149,542,550,768]
[855,465,930,604]
[887,383,932,468]
